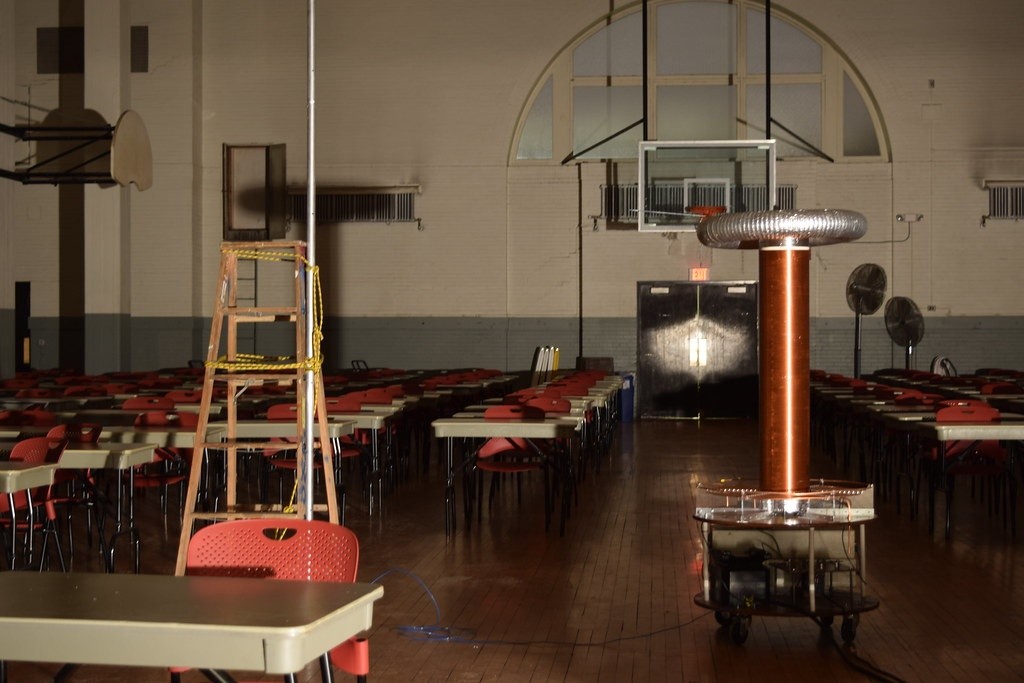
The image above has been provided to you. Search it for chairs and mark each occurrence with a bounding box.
[168,518,370,683]
[261,402,340,505]
[468,404,551,531]
[125,410,201,544]
[0,436,69,574]
[45,422,106,562]
[917,405,1016,542]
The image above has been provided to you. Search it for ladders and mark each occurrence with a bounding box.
[172,241,341,575]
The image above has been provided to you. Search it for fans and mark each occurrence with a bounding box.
[885,296,925,368]
[846,263,888,377]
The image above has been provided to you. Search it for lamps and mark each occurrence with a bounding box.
[896,213,924,223]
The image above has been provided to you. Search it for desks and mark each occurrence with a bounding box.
[808,368,1024,548]
[0,365,630,683]
